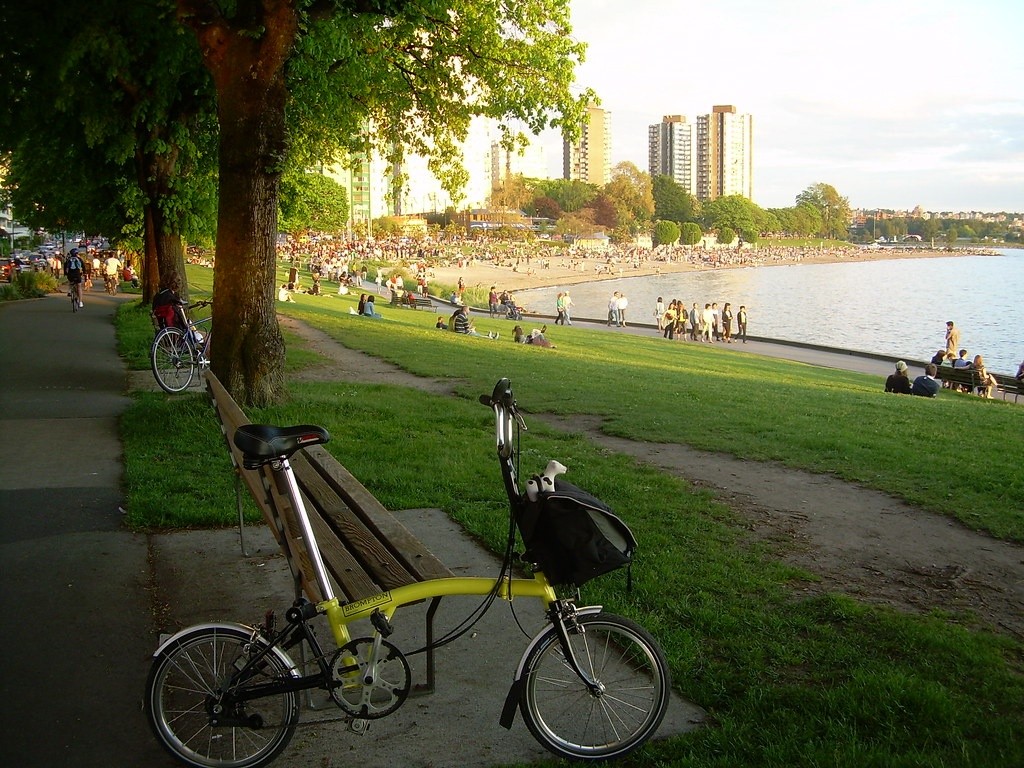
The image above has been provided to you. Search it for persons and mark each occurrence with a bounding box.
[512,325,557,348]
[489,286,508,318]
[1015,362,1024,389]
[275,232,909,305]
[884,360,913,395]
[912,363,940,398]
[43,247,140,307]
[655,297,664,333]
[357,294,384,319]
[186,248,215,268]
[607,291,628,327]
[662,299,733,343]
[734,305,748,344]
[15,256,26,272]
[931,321,994,399]
[554,290,575,325]
[436,305,499,339]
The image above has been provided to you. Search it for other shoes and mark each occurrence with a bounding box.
[488,331,491,337]
[493,332,499,339]
[79,301,84,308]
[717,338,720,341]
[684,338,686,341]
[701,339,704,342]
[709,341,714,344]
[694,339,698,341]
[743,341,747,344]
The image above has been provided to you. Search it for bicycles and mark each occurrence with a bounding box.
[141,375,674,768]
[105,274,116,295]
[68,278,83,313]
[150,288,216,394]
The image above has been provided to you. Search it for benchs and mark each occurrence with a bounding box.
[148,309,201,377]
[934,366,987,397]
[392,297,439,313]
[201,369,454,712]
[996,375,1024,403]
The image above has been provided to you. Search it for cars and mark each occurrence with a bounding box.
[0,237,107,283]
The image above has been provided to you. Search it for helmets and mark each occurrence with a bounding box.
[70,248,78,255]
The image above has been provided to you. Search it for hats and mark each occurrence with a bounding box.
[895,361,908,372]
